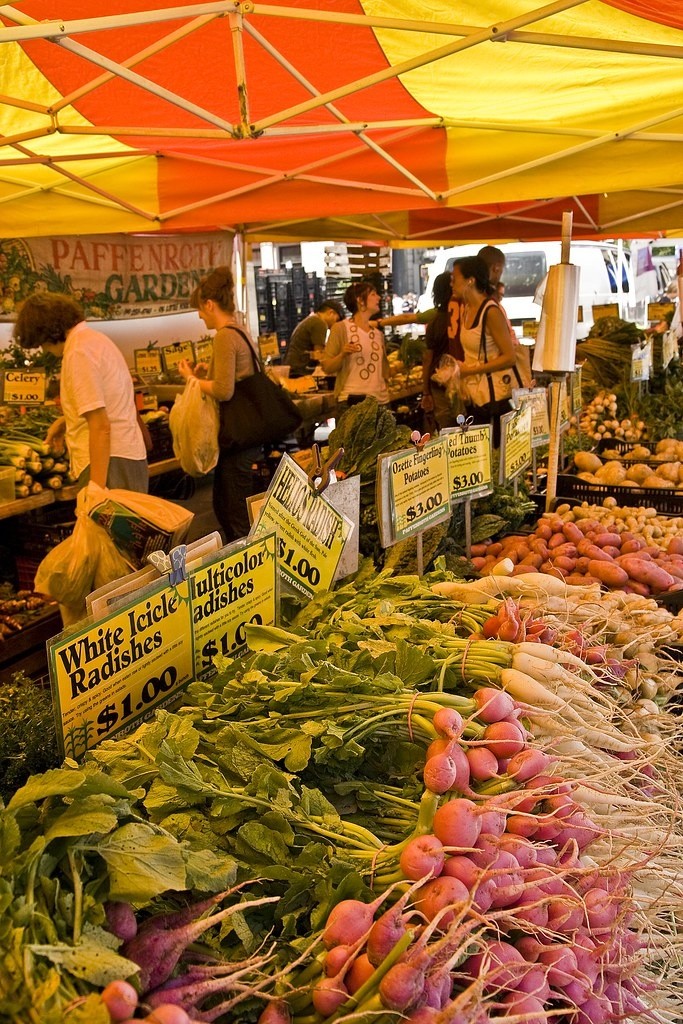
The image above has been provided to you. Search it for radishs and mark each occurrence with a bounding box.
[570,393,649,443]
[427,558,683,657]
[501,641,683,1024]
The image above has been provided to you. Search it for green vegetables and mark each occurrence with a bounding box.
[0,670,63,803]
[305,398,537,577]
[0,407,80,498]
[576,315,683,390]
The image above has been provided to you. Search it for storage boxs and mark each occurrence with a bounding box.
[18,506,75,559]
[16,555,44,598]
[499,436,683,615]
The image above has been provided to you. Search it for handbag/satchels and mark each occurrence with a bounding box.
[218,326,304,452]
[169,377,220,477]
[34,480,195,627]
[463,304,532,407]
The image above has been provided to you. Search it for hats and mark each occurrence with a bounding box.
[324,299,346,321]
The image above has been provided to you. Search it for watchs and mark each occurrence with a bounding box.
[377,318,382,328]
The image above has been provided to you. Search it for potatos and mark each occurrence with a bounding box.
[459,439,683,599]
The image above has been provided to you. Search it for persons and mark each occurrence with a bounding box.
[177,268,270,544]
[658,280,678,303]
[282,245,533,448]
[12,292,150,494]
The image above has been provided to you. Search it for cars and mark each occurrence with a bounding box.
[412,241,630,346]
